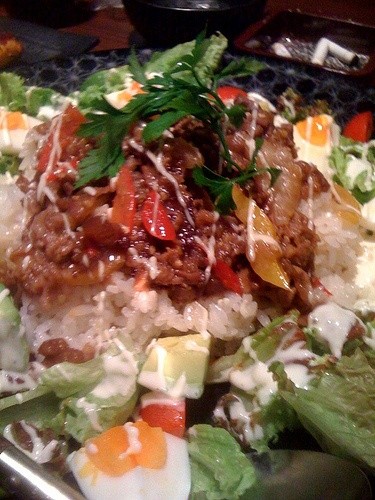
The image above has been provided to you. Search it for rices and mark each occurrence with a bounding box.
[0,31,375,500]
